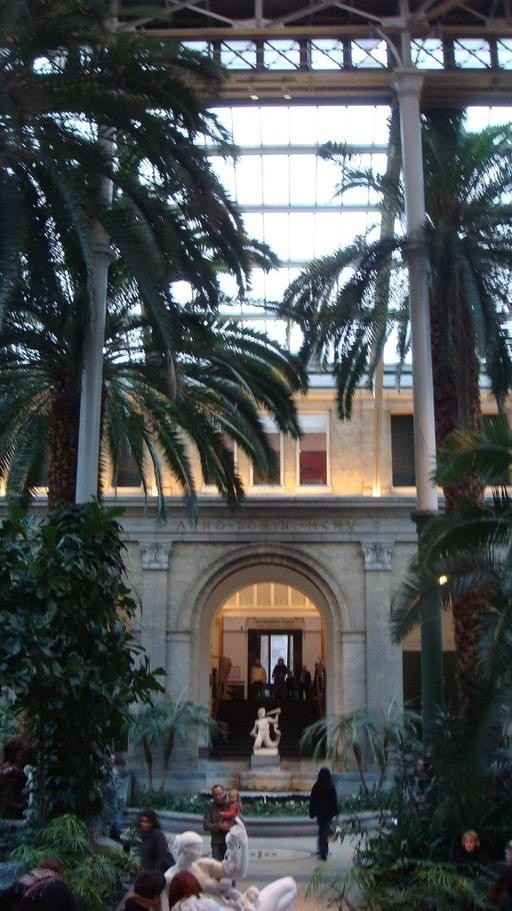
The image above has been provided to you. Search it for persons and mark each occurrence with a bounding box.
[137,809,178,875]
[308,768,341,860]
[286,670,297,699]
[166,870,226,911]
[8,854,80,911]
[451,831,490,867]
[273,670,286,709]
[298,665,312,700]
[249,658,268,698]
[243,886,259,911]
[163,817,300,911]
[217,788,246,827]
[221,832,241,875]
[120,869,167,911]
[484,839,511,911]
[216,876,245,911]
[272,658,289,699]
[248,708,284,750]
[202,784,236,890]
